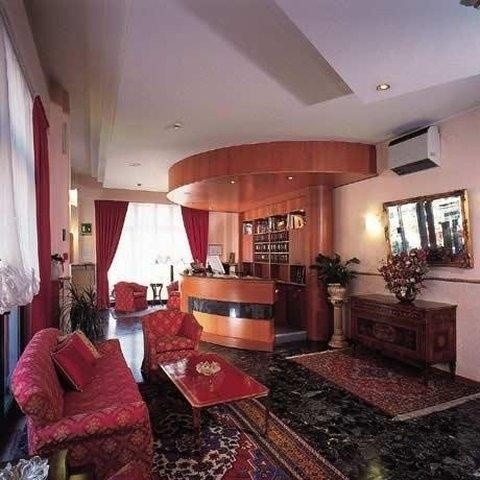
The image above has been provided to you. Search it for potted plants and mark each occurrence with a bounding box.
[307,250,361,348]
[59,281,110,344]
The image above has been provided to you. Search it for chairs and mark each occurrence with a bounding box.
[114,281,148,313]
[140,308,203,394]
[166,280,180,309]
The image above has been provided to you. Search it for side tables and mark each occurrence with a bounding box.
[150,283,164,308]
[0,446,96,480]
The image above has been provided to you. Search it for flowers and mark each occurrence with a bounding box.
[376,247,431,294]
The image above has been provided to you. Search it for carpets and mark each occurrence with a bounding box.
[12,382,349,480]
[111,304,166,320]
[286,347,480,424]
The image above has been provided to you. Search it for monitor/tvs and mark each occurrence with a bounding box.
[208,255,226,278]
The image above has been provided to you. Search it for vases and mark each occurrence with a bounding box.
[394,284,417,306]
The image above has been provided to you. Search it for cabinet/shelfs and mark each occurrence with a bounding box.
[348,292,457,387]
[239,209,307,287]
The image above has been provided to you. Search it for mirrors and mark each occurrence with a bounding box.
[383,187,475,270]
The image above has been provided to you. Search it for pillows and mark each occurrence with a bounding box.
[49,328,101,394]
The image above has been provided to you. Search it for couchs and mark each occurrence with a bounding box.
[11,325,155,480]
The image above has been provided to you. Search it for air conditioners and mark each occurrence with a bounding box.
[387,123,441,177]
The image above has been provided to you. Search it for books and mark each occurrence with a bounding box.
[294,266,306,284]
